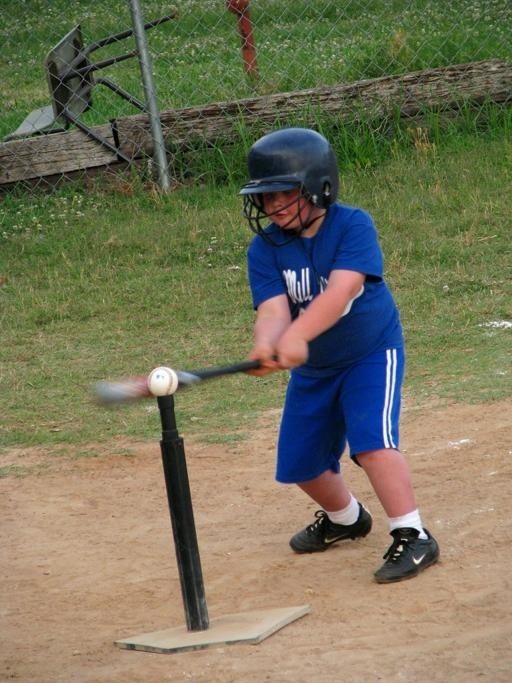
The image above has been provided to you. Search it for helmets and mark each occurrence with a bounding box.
[238,128,339,247]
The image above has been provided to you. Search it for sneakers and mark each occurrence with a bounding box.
[376,528,441,584]
[291,503,373,553]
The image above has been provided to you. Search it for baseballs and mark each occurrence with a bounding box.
[144,363,179,397]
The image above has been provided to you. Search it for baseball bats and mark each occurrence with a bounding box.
[89,351,281,410]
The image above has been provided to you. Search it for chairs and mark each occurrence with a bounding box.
[3,11,178,166]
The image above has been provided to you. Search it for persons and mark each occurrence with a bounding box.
[240,123,441,583]
